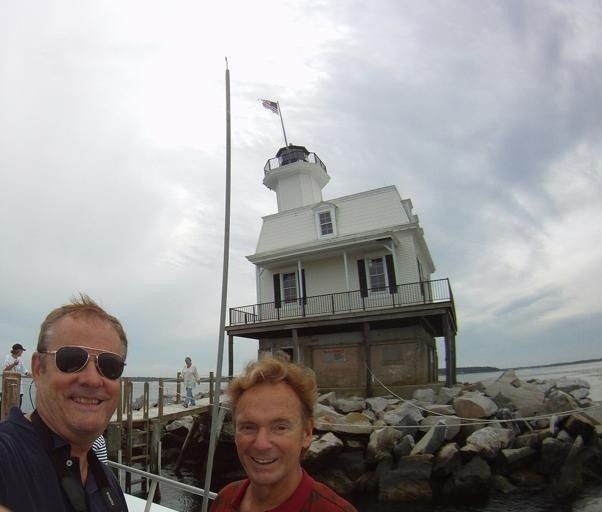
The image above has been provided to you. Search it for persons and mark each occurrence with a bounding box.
[92,435,108,464]
[3,344,32,409]
[209,358,358,512]
[179,357,200,408]
[0,294,129,512]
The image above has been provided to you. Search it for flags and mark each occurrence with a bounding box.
[262,100,278,114]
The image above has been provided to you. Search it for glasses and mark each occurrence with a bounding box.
[41,344,126,379]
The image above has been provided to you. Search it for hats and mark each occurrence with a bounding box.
[13,344,25,351]
